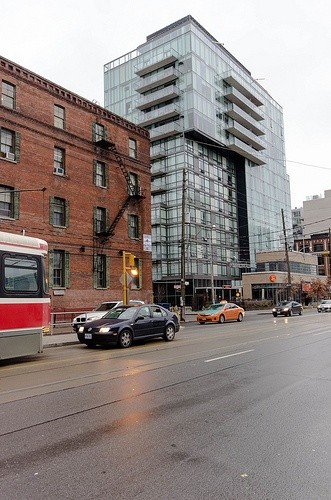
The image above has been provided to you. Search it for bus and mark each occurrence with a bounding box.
[0,230,52,360]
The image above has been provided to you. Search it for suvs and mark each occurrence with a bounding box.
[73,300,144,332]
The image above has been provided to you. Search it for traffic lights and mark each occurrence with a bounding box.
[128,254,139,277]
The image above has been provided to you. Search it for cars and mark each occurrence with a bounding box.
[272,300,303,318]
[77,303,180,348]
[197,299,245,323]
[317,300,330,313]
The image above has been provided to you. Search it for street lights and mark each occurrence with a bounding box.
[289,246,312,264]
[183,223,217,324]
